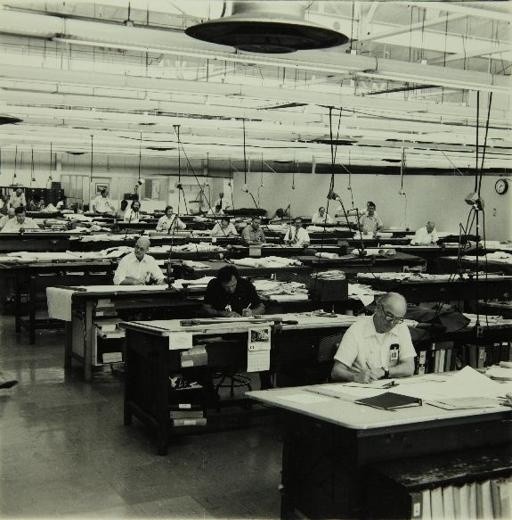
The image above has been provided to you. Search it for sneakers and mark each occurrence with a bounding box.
[0,381,19,389]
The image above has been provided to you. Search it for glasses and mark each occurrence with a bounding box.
[381,304,404,325]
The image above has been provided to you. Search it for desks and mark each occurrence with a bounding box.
[439,251,511,272]
[82,206,334,224]
[117,309,512,453]
[45,272,387,380]
[0,247,143,336]
[355,270,512,302]
[376,241,484,270]
[0,225,338,246]
[244,362,512,520]
[185,250,418,270]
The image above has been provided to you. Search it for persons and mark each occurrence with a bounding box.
[331,292,417,381]
[156,206,186,231]
[212,217,239,237]
[124,201,141,222]
[312,207,331,223]
[112,237,169,286]
[364,201,378,215]
[91,189,116,214]
[1,207,40,228]
[215,192,231,210]
[359,203,383,234]
[241,217,267,244]
[284,217,311,243]
[410,221,439,246]
[10,189,27,211]
[207,205,225,214]
[116,200,128,218]
[202,265,265,317]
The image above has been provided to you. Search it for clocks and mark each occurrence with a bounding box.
[493,179,508,193]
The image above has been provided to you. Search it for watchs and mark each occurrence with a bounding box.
[380,365,394,379]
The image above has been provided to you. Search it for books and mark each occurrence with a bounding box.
[94,319,126,338]
[411,476,511,516]
[171,402,208,427]
[417,340,511,374]
[101,351,122,364]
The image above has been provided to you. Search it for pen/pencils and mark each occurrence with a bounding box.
[246,302,252,310]
[363,360,375,374]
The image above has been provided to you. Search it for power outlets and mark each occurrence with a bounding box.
[327,192,341,201]
[465,192,484,214]
[136,181,142,187]
[175,182,183,189]
